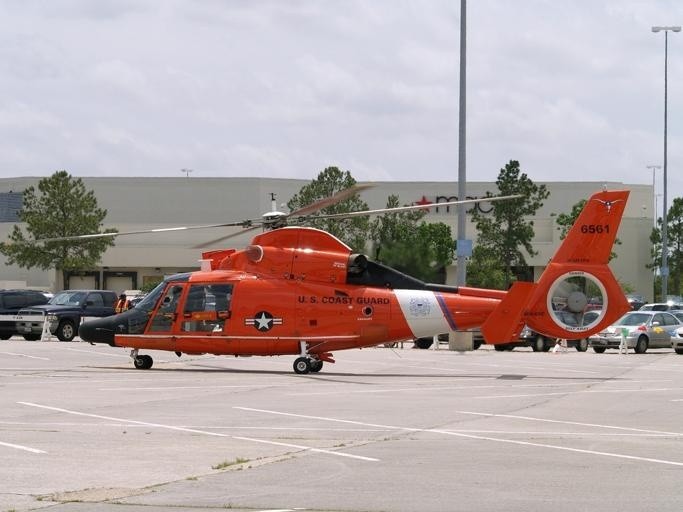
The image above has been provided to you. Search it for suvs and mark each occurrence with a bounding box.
[0,284,171,346]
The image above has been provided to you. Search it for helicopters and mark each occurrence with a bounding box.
[22,180,634,378]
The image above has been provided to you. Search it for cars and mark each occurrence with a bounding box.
[411,284,683,358]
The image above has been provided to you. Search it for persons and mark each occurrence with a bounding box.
[211,282,230,330]
[113,294,132,314]
[158,285,182,315]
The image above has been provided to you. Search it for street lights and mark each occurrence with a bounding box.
[644,24,681,304]
